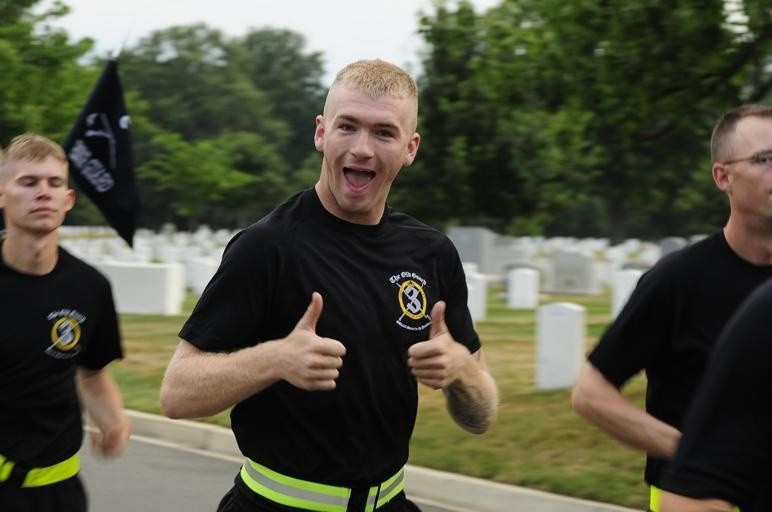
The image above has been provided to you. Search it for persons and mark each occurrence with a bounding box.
[567,102,772,512]
[0,131,131,511]
[653,271,772,512]
[160,56,499,511]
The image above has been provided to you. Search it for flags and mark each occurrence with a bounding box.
[54,60,157,260]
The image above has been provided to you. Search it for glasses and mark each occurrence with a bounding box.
[722,152,771,170]
